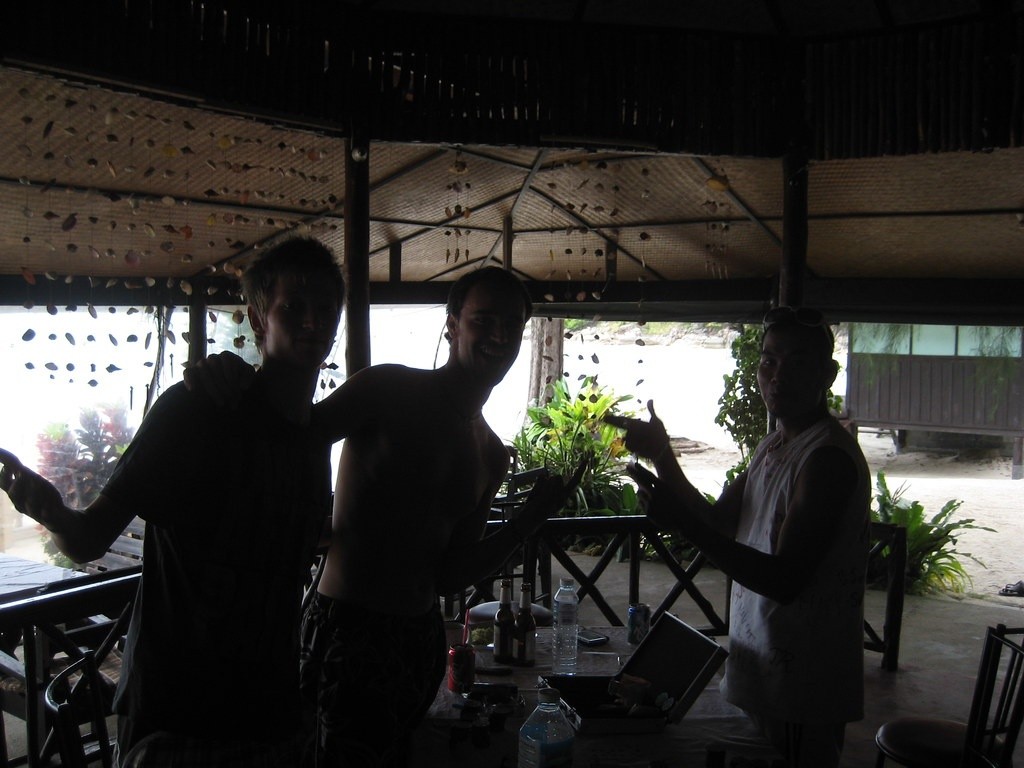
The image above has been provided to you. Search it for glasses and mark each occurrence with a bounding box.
[763,307,833,351]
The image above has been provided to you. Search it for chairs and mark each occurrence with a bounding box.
[1,595,136,768]
[875,624,1024,768]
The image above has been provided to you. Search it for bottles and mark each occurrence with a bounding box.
[552,578,579,676]
[517,688,578,768]
[493,579,516,663]
[513,583,536,665]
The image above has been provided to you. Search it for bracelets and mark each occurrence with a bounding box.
[506,516,529,547]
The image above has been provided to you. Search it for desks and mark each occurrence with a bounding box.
[404,625,792,767]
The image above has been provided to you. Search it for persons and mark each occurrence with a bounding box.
[183,268,570,768]
[0,236,345,768]
[601,305,870,768]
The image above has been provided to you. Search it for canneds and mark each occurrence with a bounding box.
[626,603,651,646]
[448,642,476,694]
